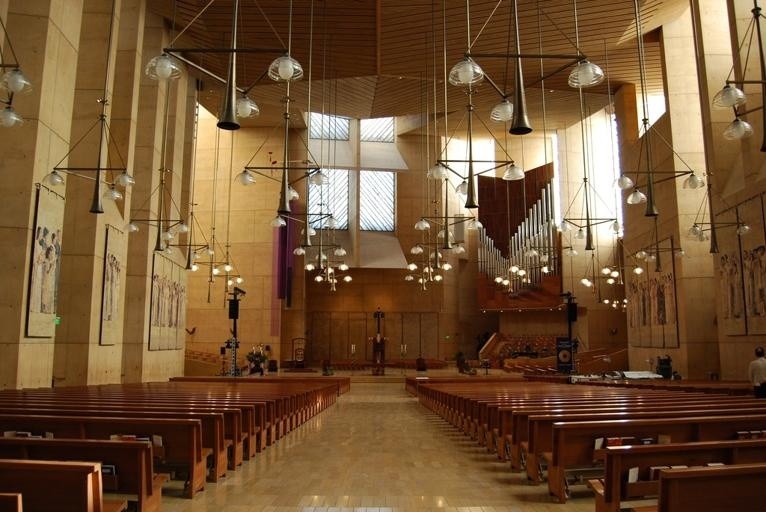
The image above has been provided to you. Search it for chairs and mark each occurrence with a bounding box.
[494,331,562,372]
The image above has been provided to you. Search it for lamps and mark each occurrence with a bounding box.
[494,331,562,372]
[0,0,353,296]
[404,0,765,313]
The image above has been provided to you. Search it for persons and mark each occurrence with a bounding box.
[748,347,766,399]
[104,253,121,321]
[719,245,766,319]
[29,226,62,315]
[152,274,185,329]
[629,273,676,328]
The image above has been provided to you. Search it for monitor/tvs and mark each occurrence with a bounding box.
[4,430,43,438]
[101,464,116,477]
[606,437,656,447]
[649,465,688,494]
[108,434,150,441]
[737,430,766,440]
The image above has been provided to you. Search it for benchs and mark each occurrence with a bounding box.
[0,370,353,510]
[406,374,766,510]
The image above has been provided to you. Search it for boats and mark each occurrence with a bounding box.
[229,300,238,319]
[568,303,577,321]
